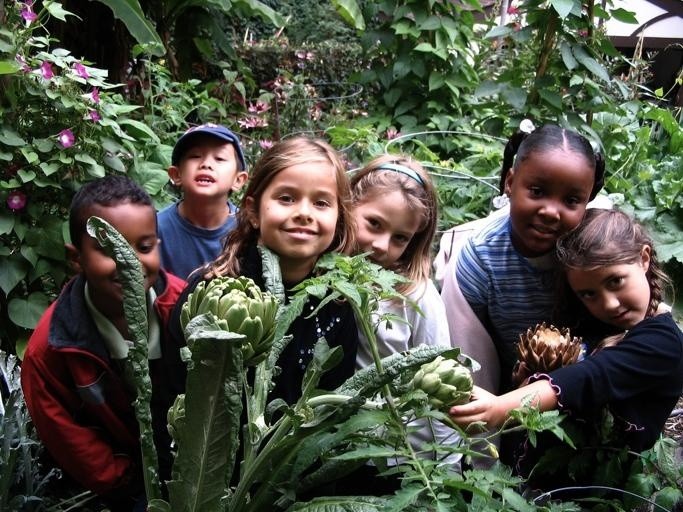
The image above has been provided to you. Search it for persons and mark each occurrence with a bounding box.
[343,153,464,477]
[153,123,245,286]
[438,119,606,478]
[153,134,360,504]
[446,204,682,498]
[18,172,191,512]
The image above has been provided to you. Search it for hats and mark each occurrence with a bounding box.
[170,121,249,174]
